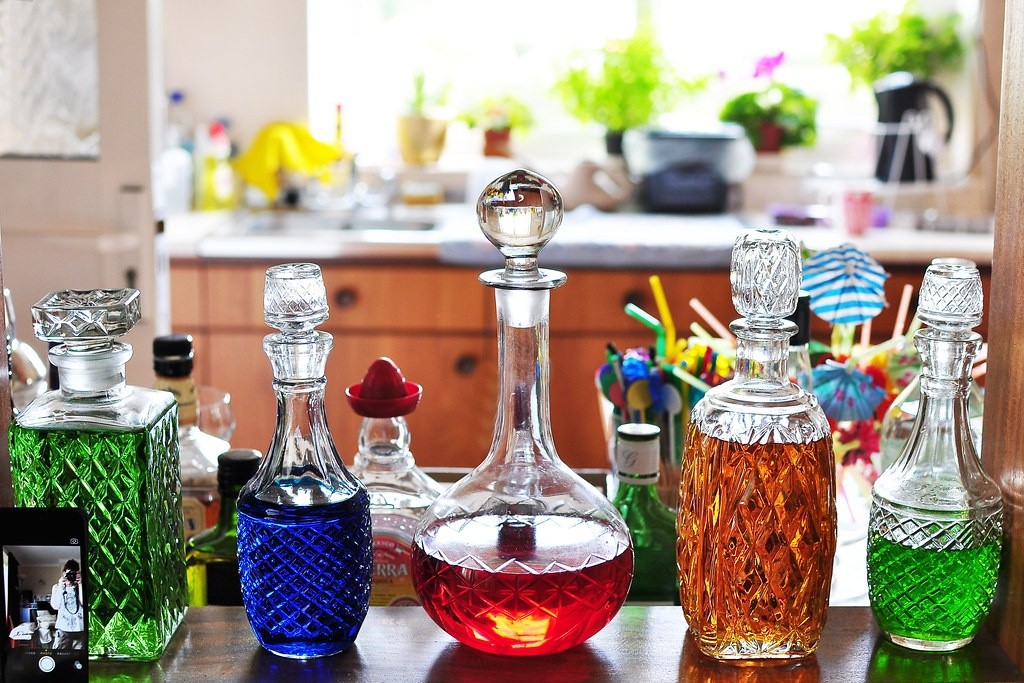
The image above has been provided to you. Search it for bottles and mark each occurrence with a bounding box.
[674,229,836,662]
[9,290,192,665]
[410,168,635,657]
[236,262,373,662]
[158,90,237,211]
[154,334,678,608]
[867,258,1002,652]
[2,291,50,416]
[880,369,985,479]
[782,290,814,395]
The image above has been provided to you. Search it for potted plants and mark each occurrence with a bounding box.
[398,71,452,168]
[458,95,532,159]
[822,0,969,186]
[552,28,716,156]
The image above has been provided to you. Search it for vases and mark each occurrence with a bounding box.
[753,125,785,154]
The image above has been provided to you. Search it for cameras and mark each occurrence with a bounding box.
[65,571,79,581]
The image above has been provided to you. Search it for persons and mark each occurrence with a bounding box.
[50,559,83,650]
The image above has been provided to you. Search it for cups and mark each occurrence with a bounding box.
[197,384,235,445]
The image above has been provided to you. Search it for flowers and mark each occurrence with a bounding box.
[720,51,816,151]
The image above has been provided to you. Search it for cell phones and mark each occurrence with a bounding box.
[0,507,90,683]
